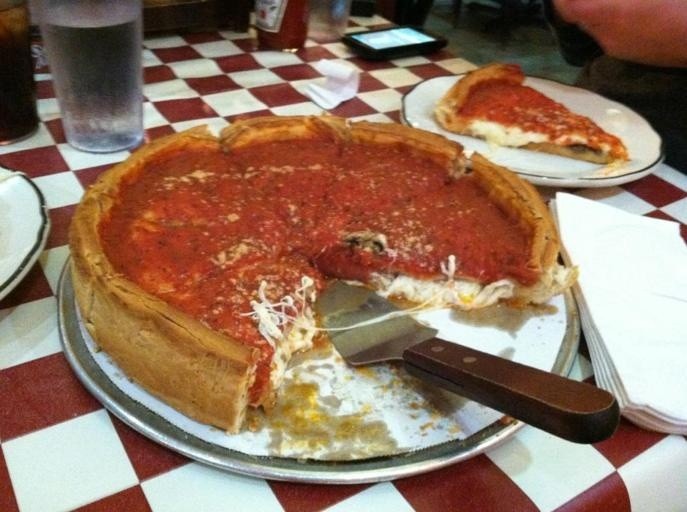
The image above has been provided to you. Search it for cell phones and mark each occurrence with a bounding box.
[339,25,450,61]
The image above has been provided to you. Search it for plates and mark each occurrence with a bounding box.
[58,255,580,482]
[401,72,666,187]
[0,166,50,301]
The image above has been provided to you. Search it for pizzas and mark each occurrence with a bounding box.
[432,62,631,174]
[66,112,581,439]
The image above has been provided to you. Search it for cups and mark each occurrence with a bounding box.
[308,2,349,42]
[0,0,39,146]
[29,1,145,154]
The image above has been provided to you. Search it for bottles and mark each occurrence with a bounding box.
[254,0,310,49]
